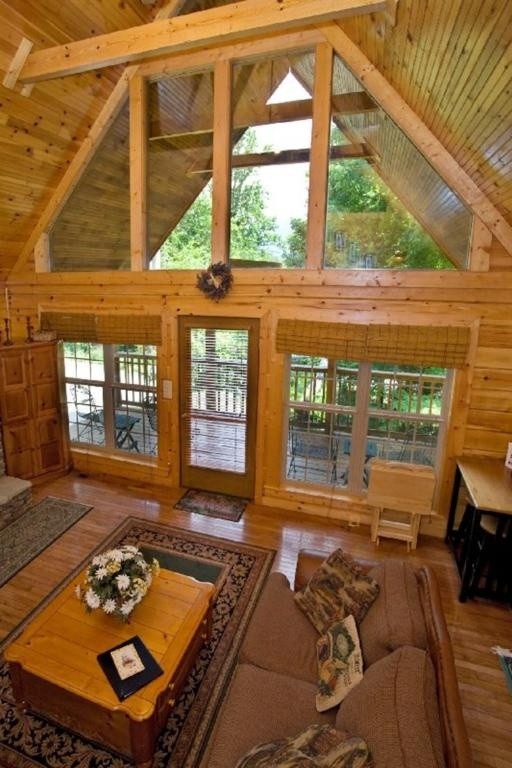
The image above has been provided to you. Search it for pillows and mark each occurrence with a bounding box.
[294,549,381,637]
[235,724,370,767]
[316,614,363,713]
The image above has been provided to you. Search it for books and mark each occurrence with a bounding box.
[96,636,164,702]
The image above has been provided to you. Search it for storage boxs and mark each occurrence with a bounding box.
[366,459,437,515]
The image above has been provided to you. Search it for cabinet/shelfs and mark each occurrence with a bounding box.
[0,338,70,486]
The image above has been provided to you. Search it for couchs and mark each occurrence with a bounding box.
[201,549,473,767]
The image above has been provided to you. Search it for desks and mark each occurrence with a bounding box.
[341,440,377,486]
[445,457,512,608]
[115,414,142,453]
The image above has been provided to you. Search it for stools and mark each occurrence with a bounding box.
[0,475,34,531]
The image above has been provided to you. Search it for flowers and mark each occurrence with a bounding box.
[75,545,160,622]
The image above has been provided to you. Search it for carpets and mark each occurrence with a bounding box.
[492,646,512,698]
[1,495,94,587]
[0,516,278,768]
[173,488,250,522]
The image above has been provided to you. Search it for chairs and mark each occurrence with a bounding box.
[144,400,158,454]
[380,432,437,465]
[287,415,340,482]
[74,386,106,444]
